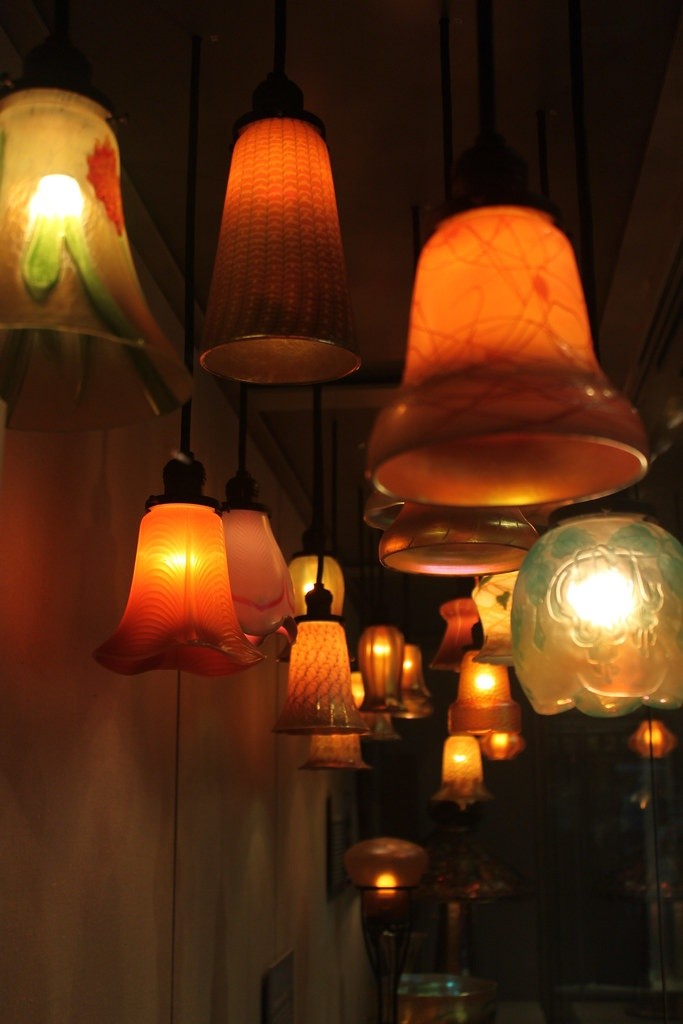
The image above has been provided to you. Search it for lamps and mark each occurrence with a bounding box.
[0,0,683,811]
[344,838,427,918]
